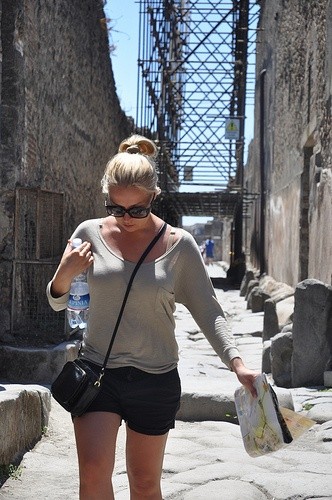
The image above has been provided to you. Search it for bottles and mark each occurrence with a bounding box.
[67,238,90,329]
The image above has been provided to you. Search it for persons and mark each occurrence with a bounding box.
[204,237,216,265]
[42,131,261,500]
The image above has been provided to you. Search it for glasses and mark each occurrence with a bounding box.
[104,192,155,218]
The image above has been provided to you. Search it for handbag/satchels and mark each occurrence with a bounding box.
[49,357,104,417]
